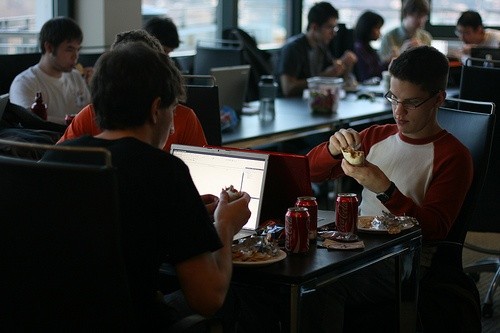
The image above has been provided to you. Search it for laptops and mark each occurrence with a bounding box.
[202,144,343,232]
[210,64,251,118]
[170,145,268,247]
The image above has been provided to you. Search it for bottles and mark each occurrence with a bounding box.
[30,92,48,120]
[258,75,279,122]
[484,54,493,66]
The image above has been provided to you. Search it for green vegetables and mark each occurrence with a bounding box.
[311,92,330,113]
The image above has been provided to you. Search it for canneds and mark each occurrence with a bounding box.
[335,193,358,233]
[64,113,77,125]
[294,197,318,240]
[285,207,310,254]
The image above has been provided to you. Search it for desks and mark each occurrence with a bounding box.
[222,86,460,150]
[233,222,423,333]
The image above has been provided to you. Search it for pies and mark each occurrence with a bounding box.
[225,185,243,204]
[340,145,364,167]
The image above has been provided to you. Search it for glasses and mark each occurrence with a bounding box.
[454,29,471,39]
[383,90,441,111]
[326,23,339,33]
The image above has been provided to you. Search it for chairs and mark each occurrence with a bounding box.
[0,139,224,333]
[179,28,271,148]
[458,57,500,232]
[402,97,496,333]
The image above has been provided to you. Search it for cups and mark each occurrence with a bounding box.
[308,76,344,114]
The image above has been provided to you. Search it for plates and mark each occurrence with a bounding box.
[358,216,418,231]
[231,248,287,264]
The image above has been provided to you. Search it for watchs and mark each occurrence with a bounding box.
[376,182,396,204]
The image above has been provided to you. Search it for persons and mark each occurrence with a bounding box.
[348,12,399,82]
[52,30,206,145]
[275,2,358,97]
[451,10,500,66]
[139,19,179,53]
[33,41,251,333]
[380,0,431,64]
[305,46,474,333]
[7,17,93,125]
